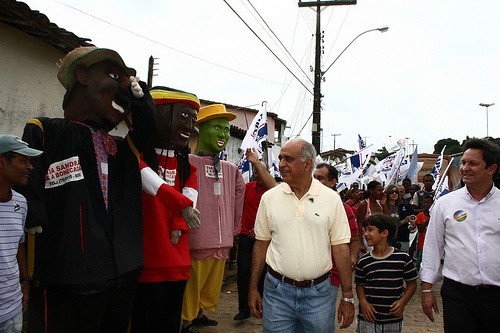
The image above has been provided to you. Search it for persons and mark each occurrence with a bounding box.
[17,46,247,333]
[233,138,436,333]
[0,135,44,333]
[419,139,500,333]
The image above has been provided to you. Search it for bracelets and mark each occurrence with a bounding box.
[421,289,433,292]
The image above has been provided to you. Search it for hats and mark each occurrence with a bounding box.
[0,134,44,157]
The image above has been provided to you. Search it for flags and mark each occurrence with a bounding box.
[236,102,452,216]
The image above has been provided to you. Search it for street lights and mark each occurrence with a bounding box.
[479,102,496,137]
[312,27,390,155]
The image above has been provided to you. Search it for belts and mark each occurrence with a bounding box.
[266,265,331,287]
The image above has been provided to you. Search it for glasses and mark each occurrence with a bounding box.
[353,185,358,187]
[390,190,399,194]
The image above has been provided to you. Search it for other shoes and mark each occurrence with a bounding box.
[233,311,251,320]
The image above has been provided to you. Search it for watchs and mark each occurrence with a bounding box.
[341,296,355,303]
